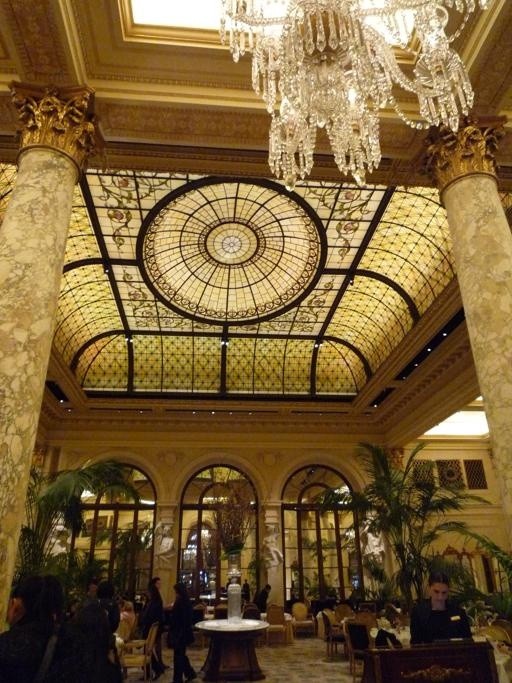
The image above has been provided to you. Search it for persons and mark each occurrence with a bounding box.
[166,583,198,683]
[255,585,271,613]
[148,577,171,671]
[119,601,135,625]
[409,572,473,646]
[76,605,122,683]
[226,578,233,590]
[86,579,97,600]
[96,582,119,633]
[1,575,96,683]
[242,579,250,605]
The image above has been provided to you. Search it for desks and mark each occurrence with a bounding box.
[194,618,270,682]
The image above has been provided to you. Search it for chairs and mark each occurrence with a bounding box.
[335,605,352,623]
[292,602,315,638]
[355,608,378,634]
[360,602,376,614]
[125,636,148,671]
[121,621,159,683]
[343,617,372,682]
[264,603,289,647]
[322,611,345,660]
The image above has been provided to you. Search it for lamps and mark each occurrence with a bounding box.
[216,0,495,193]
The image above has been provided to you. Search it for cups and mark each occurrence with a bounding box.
[261,613,267,625]
